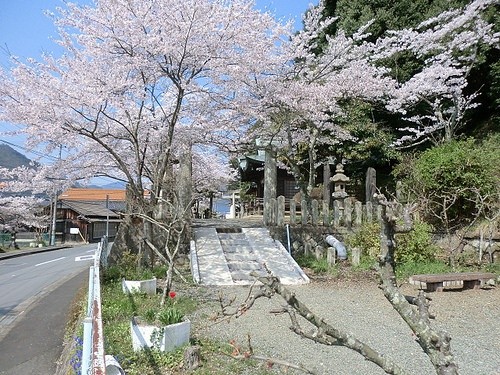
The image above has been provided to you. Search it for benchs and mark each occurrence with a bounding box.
[409,272,498,291]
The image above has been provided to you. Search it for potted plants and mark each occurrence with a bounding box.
[121,266,157,296]
[131,292,191,352]
